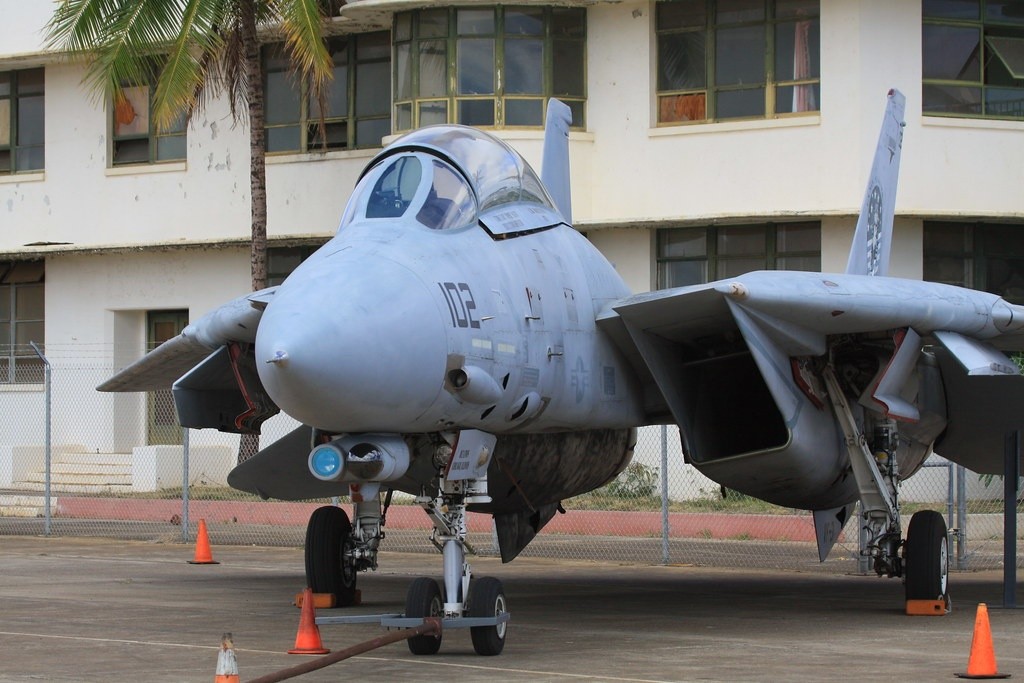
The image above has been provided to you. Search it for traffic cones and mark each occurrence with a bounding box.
[212,631,239,683]
[955,602,1013,680]
[184,519,220,565]
[285,588,331,655]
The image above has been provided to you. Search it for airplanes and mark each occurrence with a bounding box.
[93,85,1024,655]
